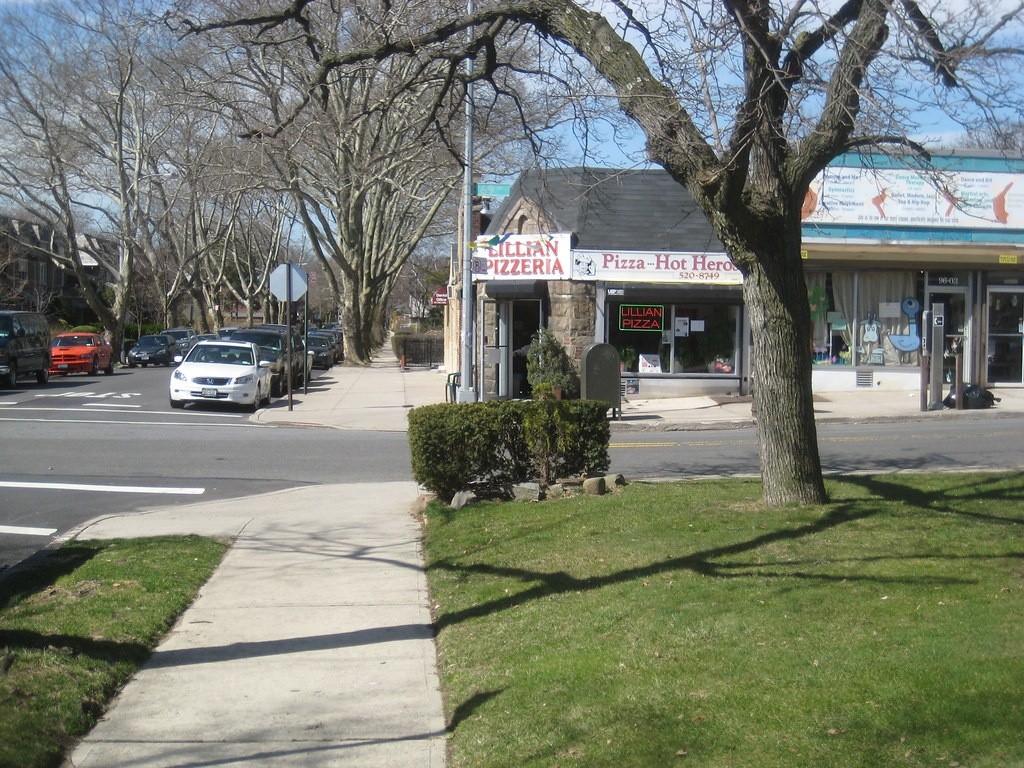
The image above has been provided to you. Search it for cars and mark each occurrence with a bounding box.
[197,334,221,342]
[169,339,271,413]
[49,332,115,376]
[127,335,180,368]
[261,322,344,391]
[229,329,289,397]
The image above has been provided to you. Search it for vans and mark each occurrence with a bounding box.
[217,327,238,341]
[0,309,52,389]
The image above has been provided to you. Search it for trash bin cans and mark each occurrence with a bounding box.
[580,342,622,423]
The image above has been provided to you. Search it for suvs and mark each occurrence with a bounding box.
[160,329,198,359]
